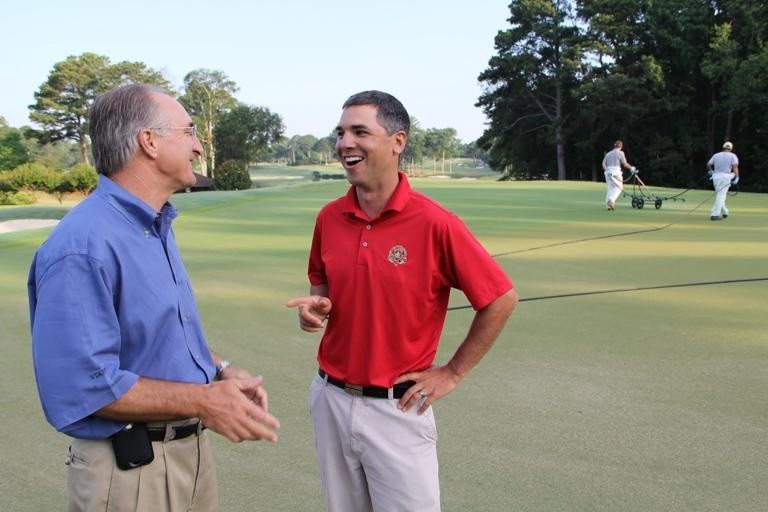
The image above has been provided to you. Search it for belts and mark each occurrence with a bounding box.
[147,421,203,443]
[317,370,411,398]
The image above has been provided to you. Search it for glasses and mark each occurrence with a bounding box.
[152,123,197,139]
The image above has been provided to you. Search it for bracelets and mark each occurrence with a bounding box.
[216,361,230,380]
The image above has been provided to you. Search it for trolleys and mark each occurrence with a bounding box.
[631,172,662,209]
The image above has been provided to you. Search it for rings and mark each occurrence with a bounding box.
[418,389,425,398]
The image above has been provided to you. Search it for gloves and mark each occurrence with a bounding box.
[630,166,636,173]
[732,176,740,185]
[708,170,714,177]
[608,170,612,177]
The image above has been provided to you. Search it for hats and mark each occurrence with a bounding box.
[722,141,733,150]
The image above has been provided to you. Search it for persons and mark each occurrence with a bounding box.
[602,141,637,211]
[286,90,518,512]
[707,142,739,220]
[27,83,281,512]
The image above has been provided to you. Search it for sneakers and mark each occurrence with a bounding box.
[711,216,722,220]
[607,200,615,211]
